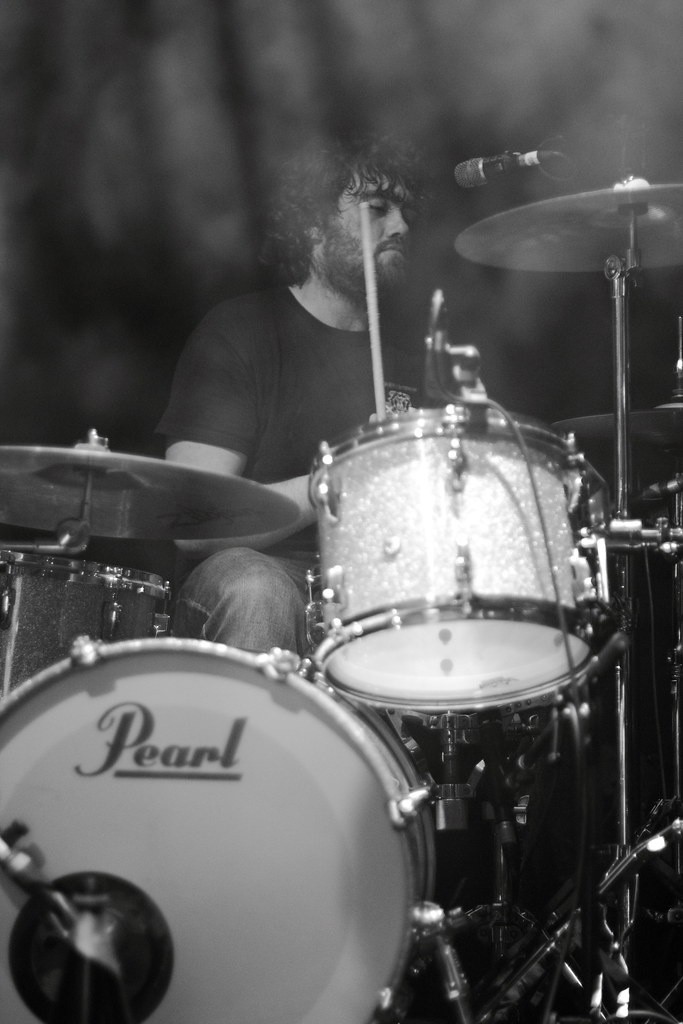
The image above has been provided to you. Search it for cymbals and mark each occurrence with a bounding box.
[453,182,683,273]
[0,445,300,542]
[551,402,683,443]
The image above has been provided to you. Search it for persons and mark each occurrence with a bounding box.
[153,133,459,656]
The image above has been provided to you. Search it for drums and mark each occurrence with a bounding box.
[301,406,611,716]
[0,548,171,697]
[0,634,440,1024]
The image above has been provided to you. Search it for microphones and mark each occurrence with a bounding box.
[0,838,118,981]
[454,150,558,189]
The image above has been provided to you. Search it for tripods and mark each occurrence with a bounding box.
[444,210,683,1024]
